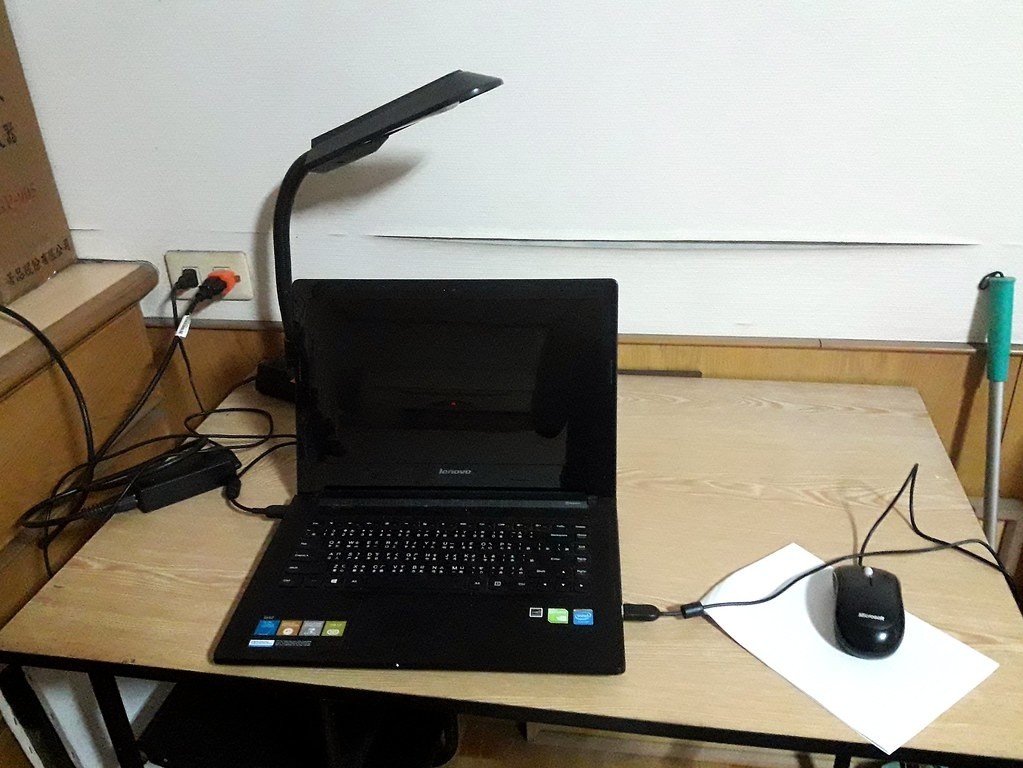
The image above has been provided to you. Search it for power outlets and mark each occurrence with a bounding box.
[164,250,253,301]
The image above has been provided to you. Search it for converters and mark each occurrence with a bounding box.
[131,447,236,515]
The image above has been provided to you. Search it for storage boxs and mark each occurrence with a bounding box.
[0,257,160,551]
[0,1,79,316]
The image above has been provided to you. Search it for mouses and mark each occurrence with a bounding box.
[830,563,905,659]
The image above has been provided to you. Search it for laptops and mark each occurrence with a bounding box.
[211,279,625,679]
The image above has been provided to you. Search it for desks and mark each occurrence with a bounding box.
[1,347,1023,766]
